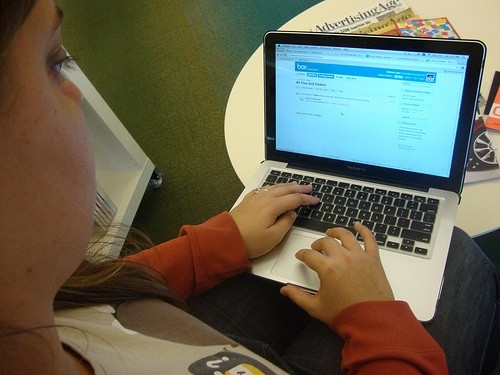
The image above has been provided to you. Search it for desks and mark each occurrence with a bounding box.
[224,0,500,239]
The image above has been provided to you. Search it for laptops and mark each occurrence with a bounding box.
[229,31,487,324]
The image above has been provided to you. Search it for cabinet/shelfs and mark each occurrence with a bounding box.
[61,46,154,264]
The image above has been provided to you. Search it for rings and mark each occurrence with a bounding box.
[254,188,269,194]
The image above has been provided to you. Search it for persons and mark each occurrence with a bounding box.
[0,0,455,375]
